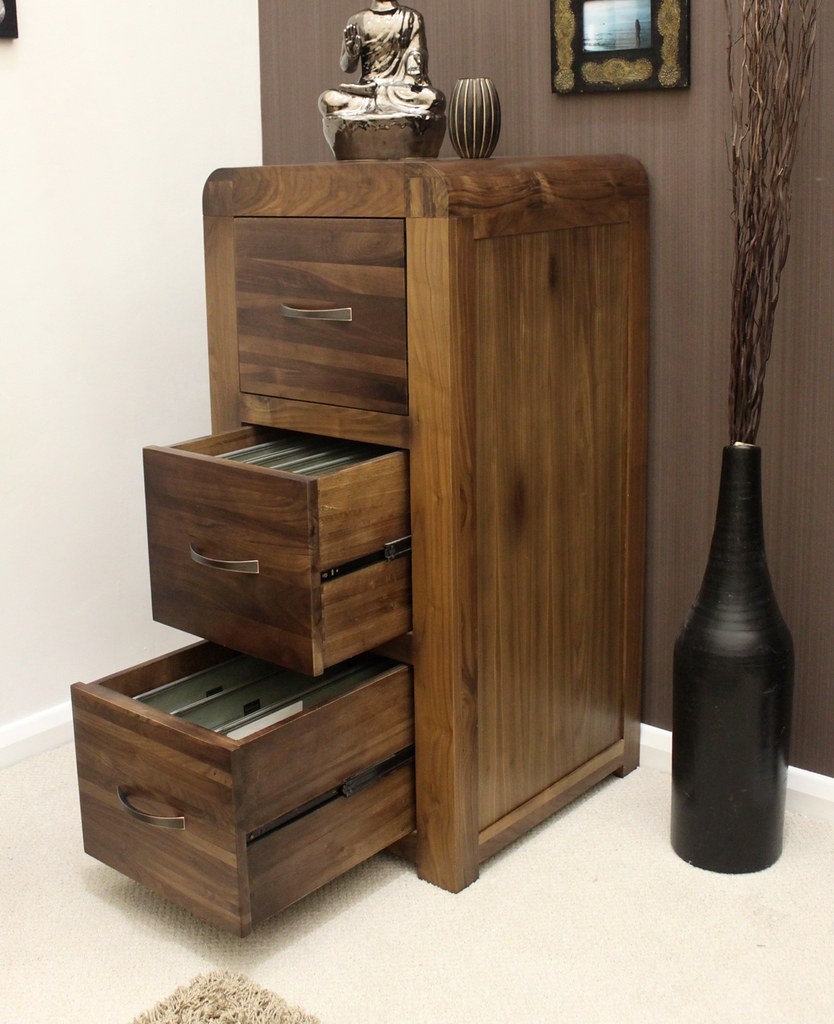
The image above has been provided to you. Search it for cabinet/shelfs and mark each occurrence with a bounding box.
[67,156,651,940]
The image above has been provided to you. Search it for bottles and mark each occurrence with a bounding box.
[450,78,501,160]
[670,441,795,874]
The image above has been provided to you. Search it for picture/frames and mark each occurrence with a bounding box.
[549,0,692,96]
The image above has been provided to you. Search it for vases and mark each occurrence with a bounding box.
[668,443,797,876]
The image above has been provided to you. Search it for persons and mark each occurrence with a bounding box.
[317,0,447,115]
